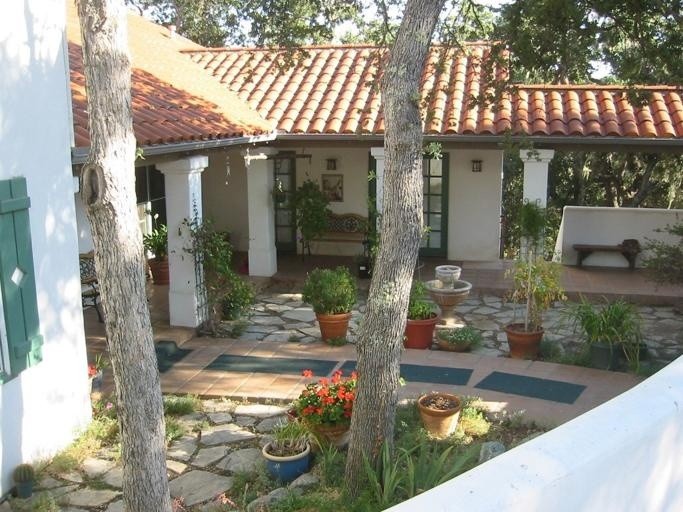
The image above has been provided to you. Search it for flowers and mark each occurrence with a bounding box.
[287,368,359,431]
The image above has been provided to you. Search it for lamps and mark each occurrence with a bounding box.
[324,158,337,170]
[471,159,484,172]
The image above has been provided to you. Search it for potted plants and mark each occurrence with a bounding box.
[13,463,35,499]
[269,171,333,244]
[261,415,325,484]
[546,290,656,376]
[142,214,258,339]
[402,278,485,352]
[499,235,568,362]
[300,264,357,344]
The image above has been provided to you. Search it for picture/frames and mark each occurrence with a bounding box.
[321,174,343,202]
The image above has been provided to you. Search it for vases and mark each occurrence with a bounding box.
[317,425,350,448]
[619,342,648,360]
[417,393,462,439]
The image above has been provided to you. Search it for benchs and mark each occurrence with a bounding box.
[78,250,150,323]
[299,212,373,261]
[572,244,642,270]
